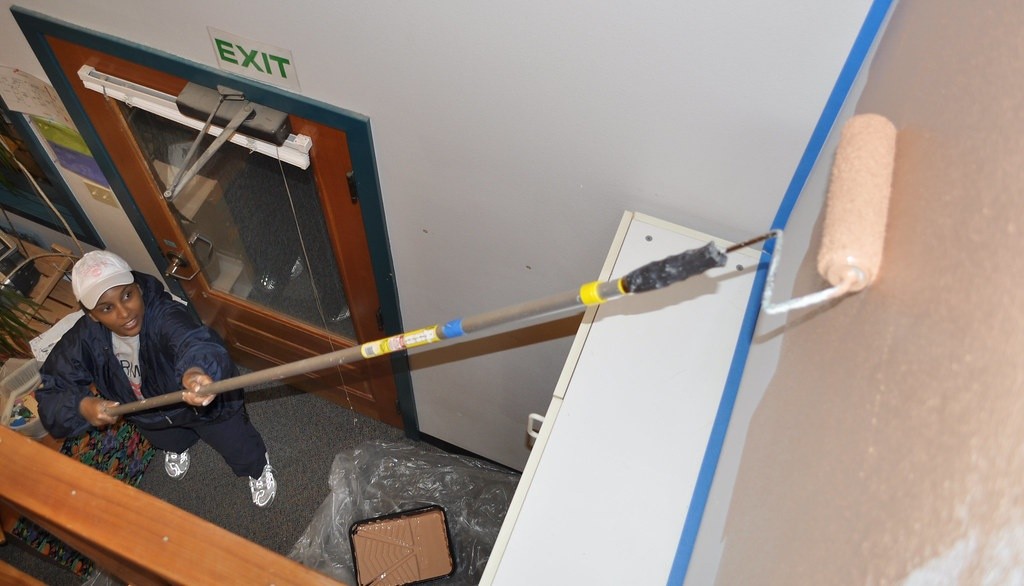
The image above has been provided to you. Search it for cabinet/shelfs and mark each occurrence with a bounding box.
[151,157,257,301]
[0,230,79,358]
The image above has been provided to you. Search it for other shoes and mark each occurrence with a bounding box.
[163,448,190,481]
[248,452,276,509]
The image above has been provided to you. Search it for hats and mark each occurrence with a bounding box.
[72,250,134,310]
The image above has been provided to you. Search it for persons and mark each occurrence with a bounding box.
[33,250,279,508]
